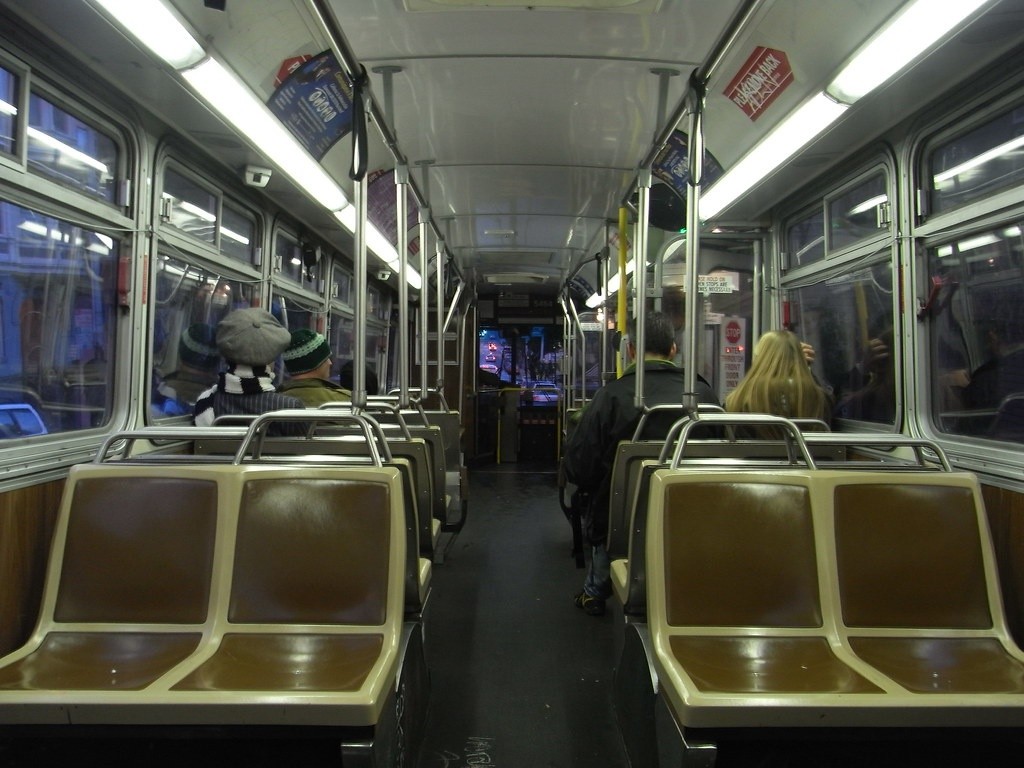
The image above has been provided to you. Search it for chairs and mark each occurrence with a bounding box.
[0,389,462,768]
[567,404,1024,768]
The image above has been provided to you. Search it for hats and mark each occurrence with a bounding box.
[283,328,332,375]
[216,308,291,365]
[177,326,221,370]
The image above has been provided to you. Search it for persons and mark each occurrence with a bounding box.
[156,320,220,412]
[190,306,320,437]
[561,311,725,615]
[721,329,834,440]
[838,327,895,425]
[939,307,1024,439]
[275,329,352,426]
[339,358,378,396]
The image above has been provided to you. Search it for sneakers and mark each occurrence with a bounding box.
[574,591,606,615]
